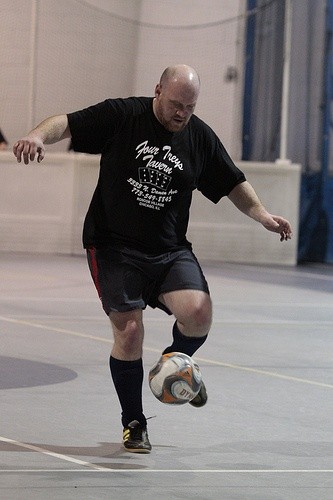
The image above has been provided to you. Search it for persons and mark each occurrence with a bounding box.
[12,63,294,453]
[0,131,8,151]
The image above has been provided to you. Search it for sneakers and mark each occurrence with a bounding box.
[121,420,152,452]
[189,380,208,407]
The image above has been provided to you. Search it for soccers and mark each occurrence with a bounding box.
[147,352,202,406]
